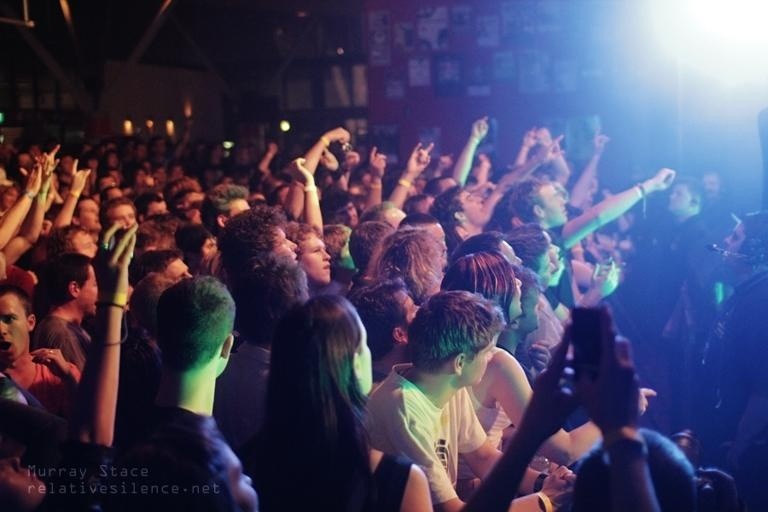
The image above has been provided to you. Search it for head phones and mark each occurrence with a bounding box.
[741,207,768,271]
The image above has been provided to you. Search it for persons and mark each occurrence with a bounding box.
[1,114,768,511]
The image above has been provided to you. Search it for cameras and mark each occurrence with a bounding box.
[107,228,133,260]
[342,142,353,153]
[696,473,720,512]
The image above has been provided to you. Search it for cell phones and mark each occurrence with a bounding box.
[571,305,606,380]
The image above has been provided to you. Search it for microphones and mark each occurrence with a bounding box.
[705,244,750,264]
